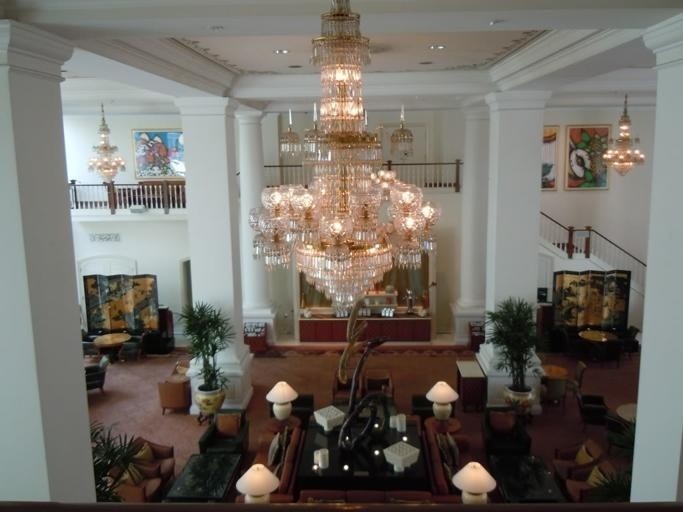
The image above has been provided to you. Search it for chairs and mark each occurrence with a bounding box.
[82,328,151,393]
[549,421,633,502]
[480,406,533,453]
[196,409,250,455]
[96,433,178,502]
[558,320,639,369]
[565,360,610,424]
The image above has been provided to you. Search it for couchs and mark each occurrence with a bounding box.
[419,422,479,502]
[234,426,306,503]
[157,358,195,416]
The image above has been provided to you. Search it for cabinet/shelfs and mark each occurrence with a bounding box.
[454,359,487,413]
[299,319,431,342]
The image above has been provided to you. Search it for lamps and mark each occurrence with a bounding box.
[602,93,647,177]
[235,463,280,504]
[424,380,460,421]
[451,460,498,506]
[87,101,127,181]
[263,379,300,421]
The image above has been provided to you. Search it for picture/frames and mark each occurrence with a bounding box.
[538,121,611,192]
[133,130,185,180]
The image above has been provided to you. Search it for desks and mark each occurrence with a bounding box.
[488,453,564,503]
[165,450,243,501]
[615,402,637,425]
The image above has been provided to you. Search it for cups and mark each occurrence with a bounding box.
[336,309,349,318]
[313,448,329,468]
[390,413,407,433]
[381,308,396,318]
[358,307,371,316]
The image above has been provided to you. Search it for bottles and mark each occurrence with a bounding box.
[300,292,305,308]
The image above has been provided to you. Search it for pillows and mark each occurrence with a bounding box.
[437,432,461,495]
[267,426,290,477]
[214,412,241,438]
[489,410,516,436]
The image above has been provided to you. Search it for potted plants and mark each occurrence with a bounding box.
[174,301,238,413]
[478,294,545,415]
